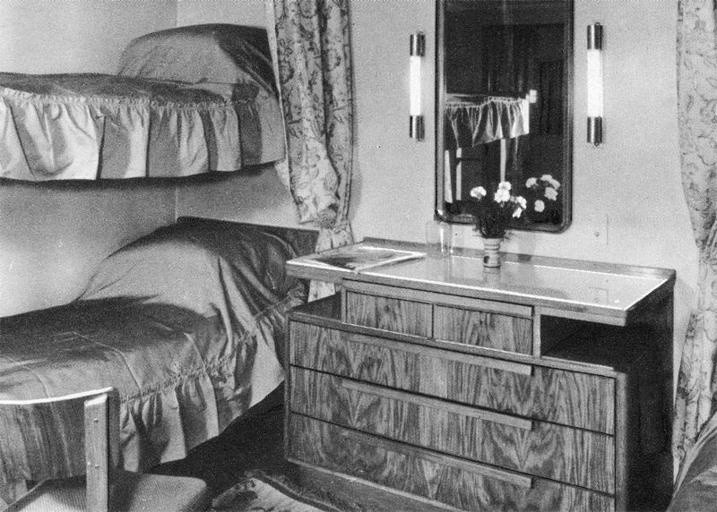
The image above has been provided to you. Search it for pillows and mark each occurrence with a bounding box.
[90,224,300,323]
[116,24,274,87]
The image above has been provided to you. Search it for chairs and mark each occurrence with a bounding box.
[0,382,209,512]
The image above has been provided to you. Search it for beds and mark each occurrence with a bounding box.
[0,27,319,511]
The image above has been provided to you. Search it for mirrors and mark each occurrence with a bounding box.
[432,2,575,236]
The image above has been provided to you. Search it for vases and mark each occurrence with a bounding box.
[472,231,510,268]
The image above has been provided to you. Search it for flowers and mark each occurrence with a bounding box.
[469,173,529,239]
[526,173,564,225]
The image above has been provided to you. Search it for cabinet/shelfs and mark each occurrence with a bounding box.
[281,234,678,509]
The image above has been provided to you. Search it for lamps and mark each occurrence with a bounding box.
[407,31,423,144]
[585,22,603,147]
